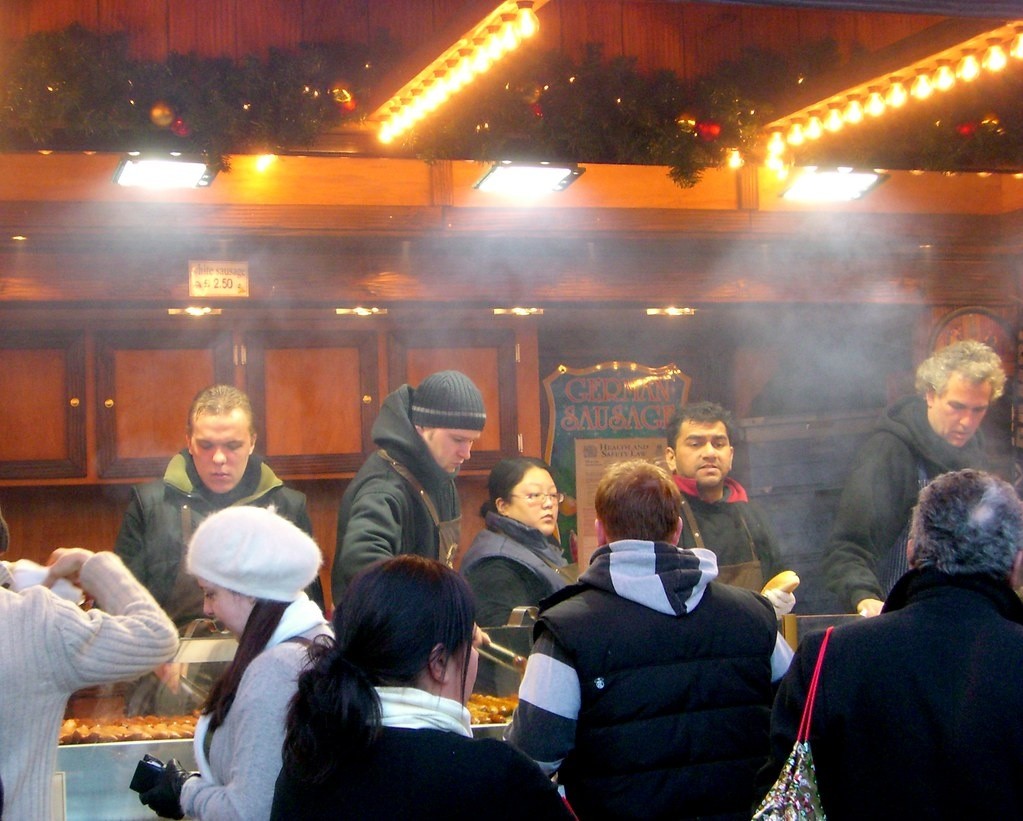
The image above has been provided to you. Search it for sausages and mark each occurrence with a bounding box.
[58,709,199,744]
[467,656,527,725]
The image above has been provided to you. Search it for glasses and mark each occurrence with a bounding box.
[510,492,565,510]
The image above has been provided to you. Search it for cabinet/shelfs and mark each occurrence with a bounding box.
[0,301,539,487]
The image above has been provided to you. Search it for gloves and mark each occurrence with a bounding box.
[138,751,191,821]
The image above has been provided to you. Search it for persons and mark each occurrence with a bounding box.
[502,461,795,821]
[818,340,1023,618]
[0,515,180,821]
[459,457,569,701]
[770,469,1023,821]
[331,369,491,650]
[138,506,335,821]
[269,555,576,821]
[92,385,325,716]
[654,401,796,633]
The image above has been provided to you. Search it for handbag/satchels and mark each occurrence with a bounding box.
[753,626,834,821]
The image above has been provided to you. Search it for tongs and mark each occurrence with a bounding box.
[474,641,525,673]
[180,670,212,699]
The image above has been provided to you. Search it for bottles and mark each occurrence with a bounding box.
[1,560,85,605]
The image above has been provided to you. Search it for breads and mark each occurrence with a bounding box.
[760,571,800,596]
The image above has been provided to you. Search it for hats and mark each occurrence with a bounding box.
[188,505,324,603]
[409,368,484,432]
